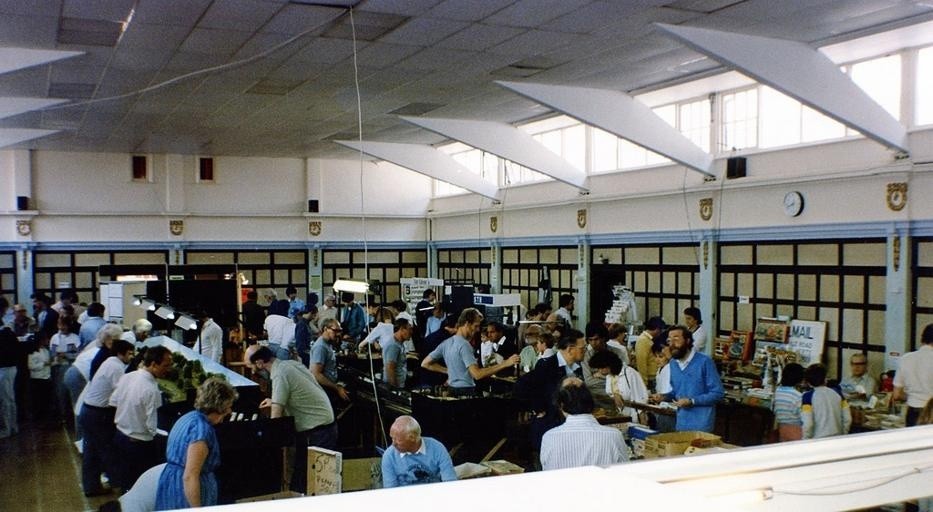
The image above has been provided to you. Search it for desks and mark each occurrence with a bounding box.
[719,385,906,447]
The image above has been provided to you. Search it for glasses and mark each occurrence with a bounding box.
[666,337,690,344]
[329,326,344,332]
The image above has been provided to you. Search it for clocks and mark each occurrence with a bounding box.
[577,210,586,228]
[782,191,804,217]
[16,220,32,236]
[170,220,183,235]
[309,221,321,236]
[699,198,712,220]
[490,217,497,232]
[886,183,908,210]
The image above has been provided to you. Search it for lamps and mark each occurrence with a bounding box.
[333,280,384,323]
[154,306,186,319]
[174,314,201,354]
[139,298,164,311]
[128,294,149,306]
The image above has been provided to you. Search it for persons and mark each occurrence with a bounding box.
[421,308,521,396]
[684,307,708,354]
[50,316,81,424]
[415,289,437,353]
[51,291,72,315]
[74,324,123,483]
[295,304,311,370]
[286,287,305,319]
[536,333,555,361]
[393,299,418,353]
[418,315,459,390]
[488,322,517,378]
[605,323,631,367]
[364,294,375,332]
[34,299,59,340]
[635,317,666,394]
[154,377,239,511]
[30,293,54,308]
[539,378,630,471]
[359,322,383,354]
[801,364,852,440]
[365,300,400,323]
[382,416,459,489]
[546,294,575,333]
[581,322,625,409]
[512,329,586,473]
[78,303,107,352]
[649,333,679,434]
[261,288,278,316]
[263,299,290,358]
[78,340,137,497]
[277,318,298,361]
[64,347,102,457]
[310,294,342,334]
[526,309,538,321]
[26,330,50,416]
[242,291,267,340]
[109,345,173,496]
[60,305,82,336]
[78,318,153,357]
[525,375,580,472]
[77,302,90,332]
[894,324,933,512]
[358,309,410,353]
[481,328,496,367]
[588,349,648,424]
[774,363,805,443]
[8,304,35,337]
[425,303,445,338]
[96,463,167,512]
[192,308,223,365]
[0,317,27,438]
[535,303,553,321]
[839,353,876,403]
[244,345,339,496]
[515,325,551,373]
[0,297,15,326]
[291,293,318,325]
[72,291,88,318]
[383,318,414,391]
[648,324,725,435]
[309,319,351,420]
[340,292,366,352]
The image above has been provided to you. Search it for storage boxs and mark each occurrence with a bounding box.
[645,431,722,457]
[453,462,491,479]
